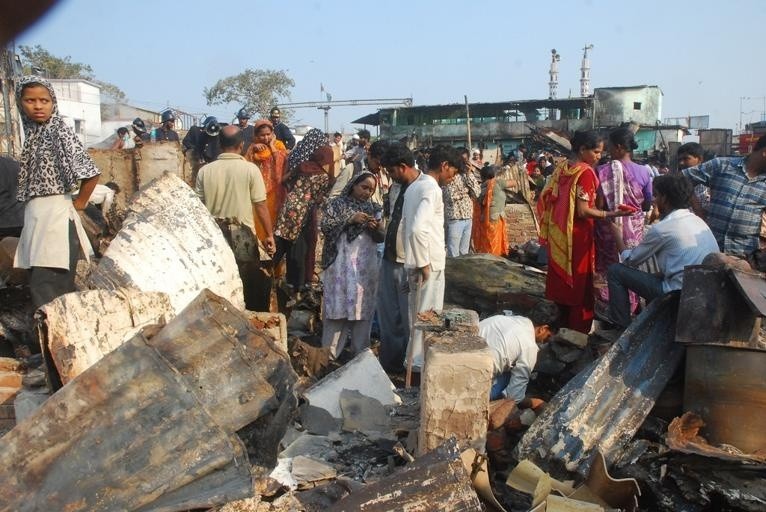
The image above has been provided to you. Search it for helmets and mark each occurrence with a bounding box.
[237,109,251,119]
[132,118,146,133]
[202,116,216,126]
[159,111,175,124]
[270,107,280,117]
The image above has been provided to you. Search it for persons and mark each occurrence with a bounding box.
[71,183,122,239]
[14,73,103,392]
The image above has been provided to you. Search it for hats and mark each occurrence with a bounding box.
[206,119,222,137]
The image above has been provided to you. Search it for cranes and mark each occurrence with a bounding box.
[265,82,417,136]
